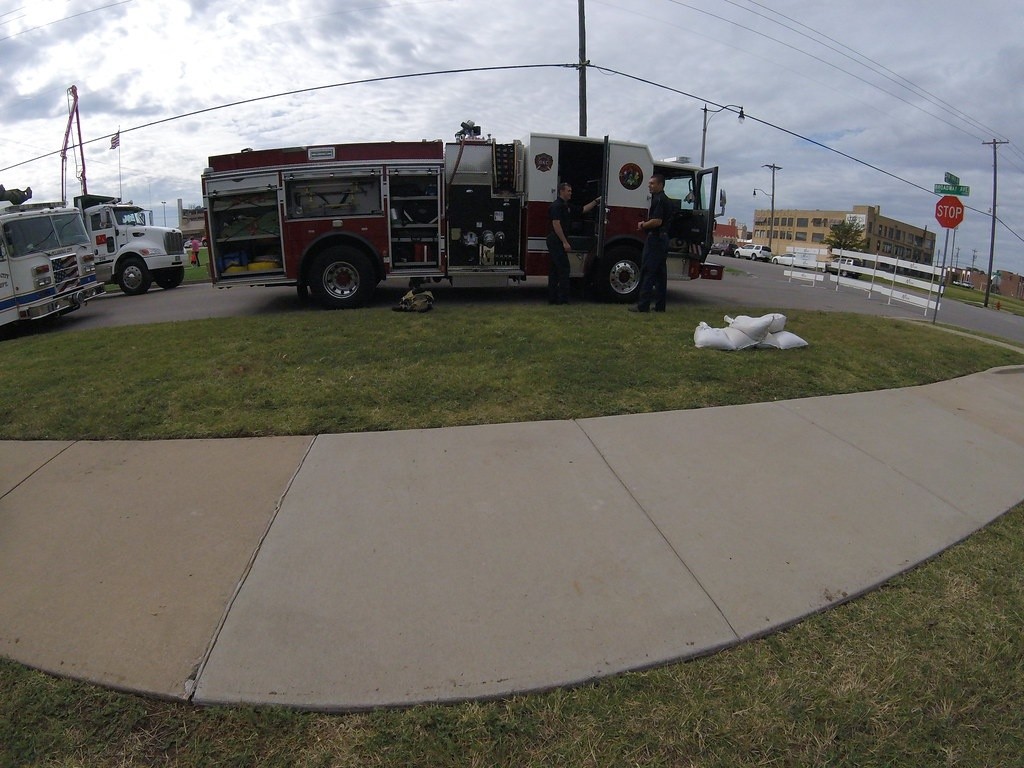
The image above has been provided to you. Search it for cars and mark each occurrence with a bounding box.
[772,253,796,265]
[708,242,739,258]
[183,236,206,248]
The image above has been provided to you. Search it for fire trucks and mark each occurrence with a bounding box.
[199,121,727,305]
[0,184,108,335]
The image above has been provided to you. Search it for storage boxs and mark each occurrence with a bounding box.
[700,262,725,280]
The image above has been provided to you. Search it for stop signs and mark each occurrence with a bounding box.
[935,195,965,229]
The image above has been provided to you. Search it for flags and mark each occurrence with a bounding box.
[110,131,120,151]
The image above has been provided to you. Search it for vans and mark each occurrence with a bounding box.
[832,258,862,278]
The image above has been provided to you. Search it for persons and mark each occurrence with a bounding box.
[626,173,679,316]
[540,183,602,306]
[189,236,201,270]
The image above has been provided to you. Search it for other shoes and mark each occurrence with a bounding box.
[628,305,641,312]
[651,305,663,313]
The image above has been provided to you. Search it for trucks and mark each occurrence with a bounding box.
[71,194,190,295]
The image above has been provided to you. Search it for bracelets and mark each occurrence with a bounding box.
[640,222,644,228]
[594,198,598,203]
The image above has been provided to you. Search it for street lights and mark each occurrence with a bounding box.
[700,104,746,170]
[753,163,783,247]
[161,201,167,228]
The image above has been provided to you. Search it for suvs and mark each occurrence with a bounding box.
[734,243,772,263]
